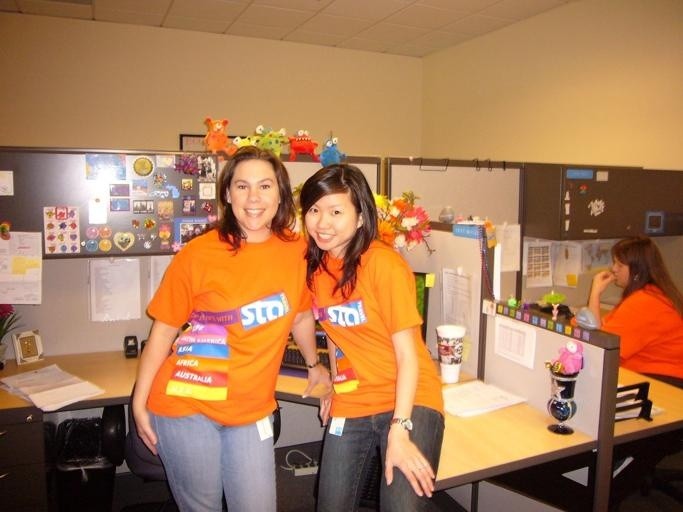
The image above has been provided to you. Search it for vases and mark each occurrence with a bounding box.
[0,304,24,368]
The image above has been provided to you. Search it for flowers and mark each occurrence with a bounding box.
[371,190,436,257]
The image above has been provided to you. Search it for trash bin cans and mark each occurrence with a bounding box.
[54,417,116,512]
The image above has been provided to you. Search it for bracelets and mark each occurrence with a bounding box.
[308,361,320,368]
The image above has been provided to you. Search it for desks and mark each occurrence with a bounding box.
[0,348,596,512]
[614,368,683,448]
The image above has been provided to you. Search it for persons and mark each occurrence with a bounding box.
[587,236,683,391]
[303,164,445,512]
[132,146,331,512]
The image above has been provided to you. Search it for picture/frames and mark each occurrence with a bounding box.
[12,329,46,365]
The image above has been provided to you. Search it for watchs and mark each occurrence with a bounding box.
[391,418,413,431]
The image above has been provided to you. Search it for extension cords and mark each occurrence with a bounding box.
[292,461,318,476]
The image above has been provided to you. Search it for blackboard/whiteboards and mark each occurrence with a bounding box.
[384,158,525,276]
[216,151,381,232]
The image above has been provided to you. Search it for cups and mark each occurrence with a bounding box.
[436,326,466,383]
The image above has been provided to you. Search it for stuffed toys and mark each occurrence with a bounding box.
[202,116,347,167]
[551,341,584,375]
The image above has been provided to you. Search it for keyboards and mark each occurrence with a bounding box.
[281,348,329,371]
